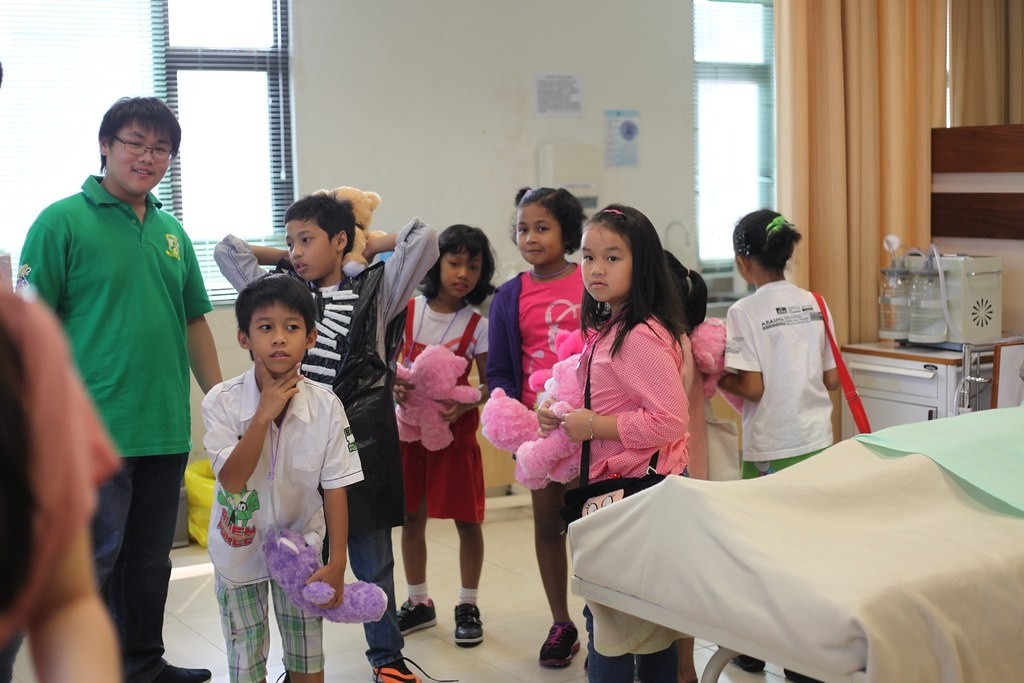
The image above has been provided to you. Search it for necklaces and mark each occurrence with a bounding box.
[529,262,571,279]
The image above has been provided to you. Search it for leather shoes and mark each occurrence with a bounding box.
[151,665,212,683]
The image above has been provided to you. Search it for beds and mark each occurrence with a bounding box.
[566,409,1024,683]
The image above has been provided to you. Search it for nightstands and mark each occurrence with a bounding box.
[841,341,996,441]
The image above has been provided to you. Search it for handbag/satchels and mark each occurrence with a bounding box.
[558,474,666,533]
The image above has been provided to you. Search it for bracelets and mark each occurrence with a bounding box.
[589,414,603,451]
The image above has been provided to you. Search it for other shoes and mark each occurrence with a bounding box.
[718,645,765,671]
[784,669,824,683]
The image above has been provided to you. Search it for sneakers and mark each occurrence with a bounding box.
[539,622,580,666]
[396,598,437,636]
[373,659,422,683]
[454,603,484,642]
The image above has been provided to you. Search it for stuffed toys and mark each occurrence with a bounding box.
[691,316,742,414]
[528,330,585,394]
[311,185,388,277]
[261,528,388,624]
[480,388,551,489]
[518,353,585,482]
[393,344,481,451]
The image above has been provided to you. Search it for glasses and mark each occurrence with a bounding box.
[114,135,174,160]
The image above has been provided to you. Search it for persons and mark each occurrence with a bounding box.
[0,288,125,683]
[16,94,223,683]
[200,188,839,683]
